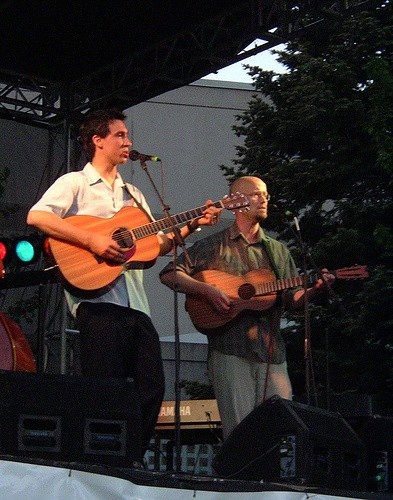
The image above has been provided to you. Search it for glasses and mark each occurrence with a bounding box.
[246,191,271,201]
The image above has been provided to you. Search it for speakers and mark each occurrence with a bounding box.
[211,395,370,491]
[0,368,147,467]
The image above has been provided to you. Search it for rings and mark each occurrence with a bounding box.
[213,216,217,218]
[215,305,218,309]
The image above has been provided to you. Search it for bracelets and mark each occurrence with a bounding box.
[186,221,194,233]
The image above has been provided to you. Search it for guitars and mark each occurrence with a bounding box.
[185,263,373,333]
[44,190,252,300]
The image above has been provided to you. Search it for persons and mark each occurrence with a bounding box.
[26,109,220,467]
[158,177,334,441]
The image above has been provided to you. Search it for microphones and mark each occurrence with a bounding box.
[130,150,159,162]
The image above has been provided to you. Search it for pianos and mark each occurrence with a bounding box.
[158,395,226,476]
